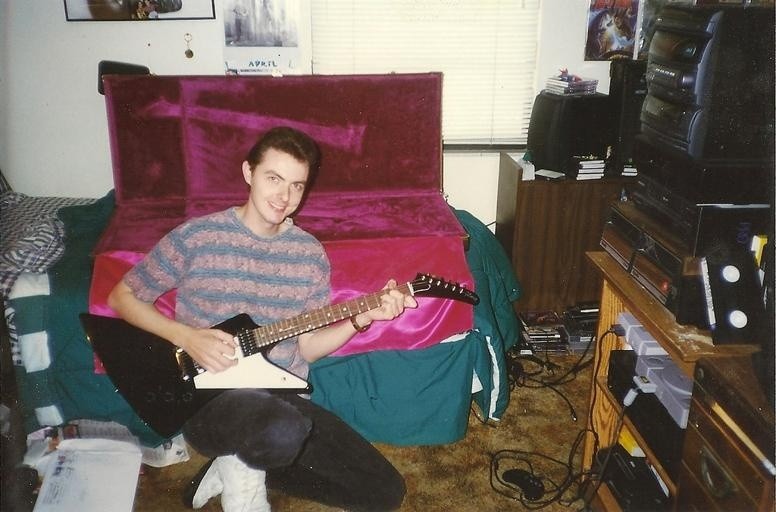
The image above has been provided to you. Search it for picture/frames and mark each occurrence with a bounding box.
[63,0,217,22]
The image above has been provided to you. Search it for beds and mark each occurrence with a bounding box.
[0,199,522,445]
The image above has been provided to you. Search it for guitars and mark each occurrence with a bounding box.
[79,273,480,440]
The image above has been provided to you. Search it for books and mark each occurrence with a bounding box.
[546,77,599,96]
[566,155,605,182]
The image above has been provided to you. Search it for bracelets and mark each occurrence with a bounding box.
[350,315,370,334]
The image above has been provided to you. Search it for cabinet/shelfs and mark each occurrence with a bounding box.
[586,250,776,512]
[494,151,636,317]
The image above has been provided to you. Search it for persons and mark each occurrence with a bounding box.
[108,127,419,512]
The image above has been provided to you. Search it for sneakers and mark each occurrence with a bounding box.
[184,453,272,512]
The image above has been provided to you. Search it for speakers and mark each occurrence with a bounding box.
[527,89,613,175]
